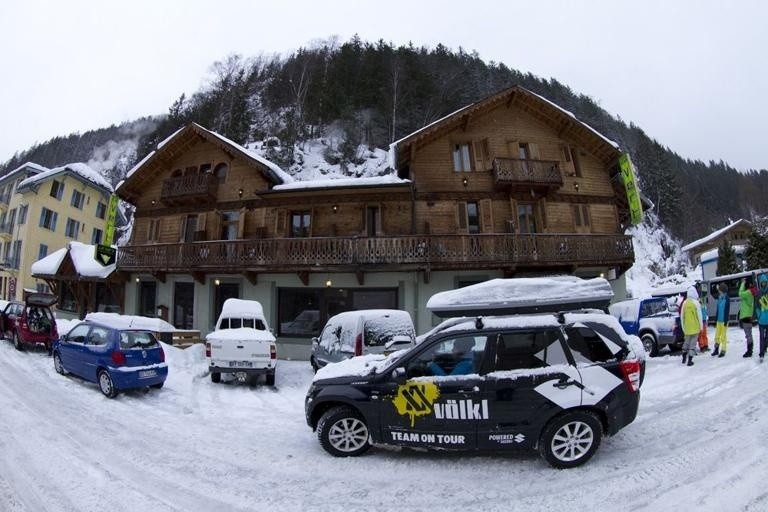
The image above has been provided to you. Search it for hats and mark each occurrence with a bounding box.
[719,285,728,292]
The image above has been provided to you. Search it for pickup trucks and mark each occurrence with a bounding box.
[206,304,278,386]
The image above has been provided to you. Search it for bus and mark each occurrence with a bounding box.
[697,269,768,324]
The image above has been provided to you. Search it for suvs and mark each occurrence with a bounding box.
[298,317,646,464]
[0,291,59,353]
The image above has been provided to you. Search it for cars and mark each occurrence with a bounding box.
[51,316,168,396]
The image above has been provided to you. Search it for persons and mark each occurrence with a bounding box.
[749,282,758,297]
[755,274,768,362]
[695,283,710,352]
[710,282,730,357]
[680,287,703,366]
[738,278,756,357]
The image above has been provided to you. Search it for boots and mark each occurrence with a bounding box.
[682,345,765,366]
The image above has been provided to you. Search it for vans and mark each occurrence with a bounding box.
[607,295,689,351]
[304,309,417,364]
